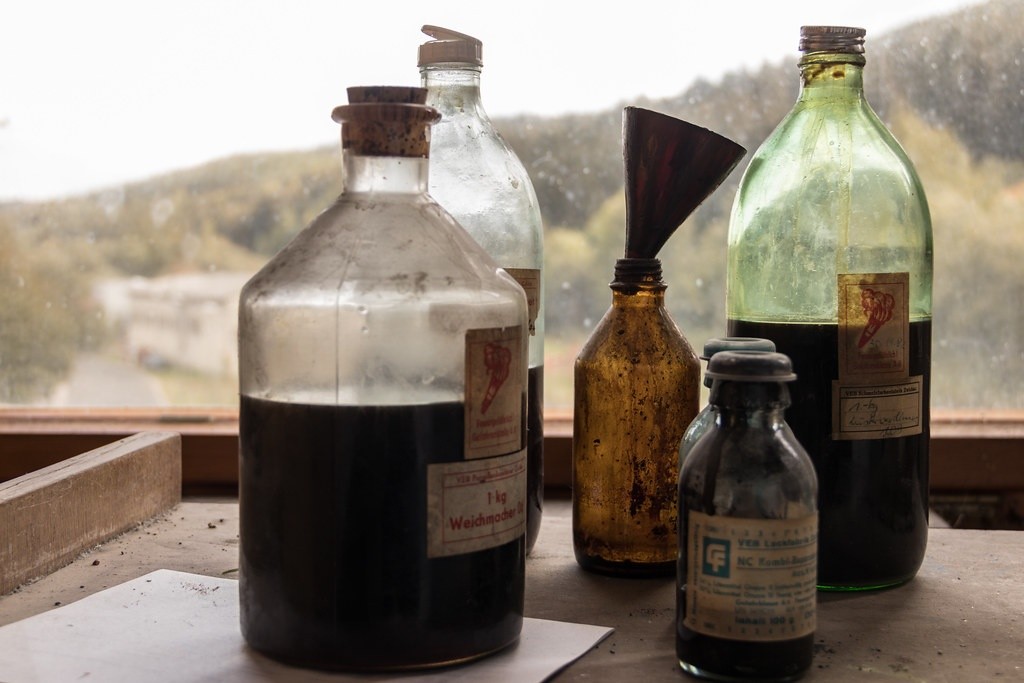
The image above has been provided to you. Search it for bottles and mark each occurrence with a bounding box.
[679,337,776,472]
[238,86,526,674]
[677,352,821,683]
[574,258,700,581]
[418,25,545,560]
[730,27,933,590]
